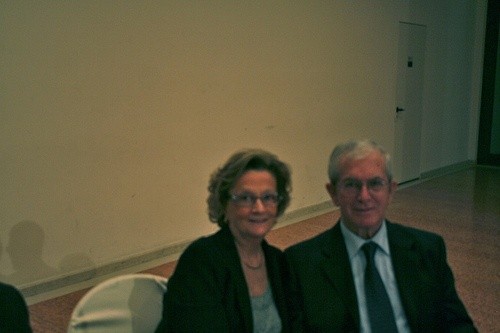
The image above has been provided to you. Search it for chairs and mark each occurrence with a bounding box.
[71,274,168,333]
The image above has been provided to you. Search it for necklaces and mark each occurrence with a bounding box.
[235,242,263,269]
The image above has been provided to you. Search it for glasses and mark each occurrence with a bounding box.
[227,191,285,209]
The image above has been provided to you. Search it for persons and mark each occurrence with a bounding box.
[155,147,308,333]
[0,282,33,333]
[282,138,479,333]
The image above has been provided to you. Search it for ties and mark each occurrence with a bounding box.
[360,243,400,333]
